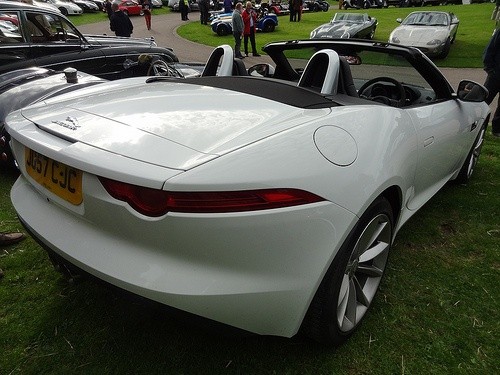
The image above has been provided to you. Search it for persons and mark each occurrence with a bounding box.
[104,0,112,20]
[211,0,236,13]
[288,0,303,22]
[482,22,500,138]
[241,1,261,57]
[232,2,245,59]
[17,0,52,37]
[110,3,133,37]
[198,0,209,24]
[179,0,189,21]
[338,0,343,10]
[141,0,151,30]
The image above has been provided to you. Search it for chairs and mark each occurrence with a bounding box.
[337,56,360,97]
[232,58,247,75]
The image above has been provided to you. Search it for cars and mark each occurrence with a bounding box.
[27,0,106,16]
[113,0,151,16]
[0,0,179,81]
[151,0,331,36]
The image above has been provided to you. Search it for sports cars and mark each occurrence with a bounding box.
[0,61,202,162]
[388,11,460,58]
[4,38,491,347]
[310,13,378,39]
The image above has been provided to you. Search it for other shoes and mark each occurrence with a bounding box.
[253,53,261,56]
[148,28,151,30]
[235,53,245,59]
[245,54,248,56]
